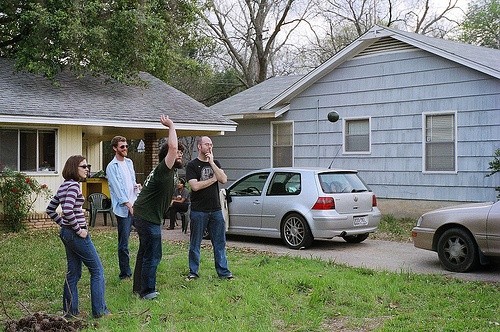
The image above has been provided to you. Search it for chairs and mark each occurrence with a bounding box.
[270,182,287,195]
[87,192,114,226]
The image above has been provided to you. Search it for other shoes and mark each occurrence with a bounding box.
[167,227,174,230]
[229,276,235,280]
[121,275,132,283]
[188,275,195,281]
[144,292,159,299]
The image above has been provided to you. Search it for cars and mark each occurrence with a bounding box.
[412,200,500,273]
[202,167,382,250]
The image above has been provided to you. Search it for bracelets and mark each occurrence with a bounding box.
[77,230,82,236]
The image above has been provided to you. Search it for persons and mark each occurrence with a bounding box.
[131,113,186,299]
[45,156,116,317]
[186,137,235,280]
[104,136,140,278]
[167,178,189,233]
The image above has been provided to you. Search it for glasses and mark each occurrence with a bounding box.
[177,151,183,156]
[78,165,91,168]
[200,143,213,148]
[116,145,129,149]
